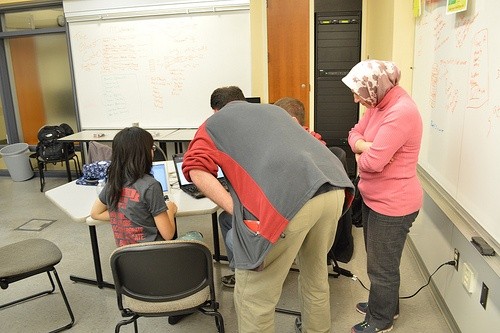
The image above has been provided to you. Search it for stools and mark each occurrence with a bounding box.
[36,155,82,193]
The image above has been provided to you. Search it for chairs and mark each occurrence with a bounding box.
[290,172,361,277]
[0,239,76,333]
[108,240,226,333]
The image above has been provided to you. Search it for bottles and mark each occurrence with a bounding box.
[96,180,105,197]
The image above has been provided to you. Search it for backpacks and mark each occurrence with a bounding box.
[36,123,76,161]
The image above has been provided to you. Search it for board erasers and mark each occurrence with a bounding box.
[470,237,495,256]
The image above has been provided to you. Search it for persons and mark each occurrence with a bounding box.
[90,127,204,325]
[341,60,424,333]
[221,98,326,288]
[182,86,355,333]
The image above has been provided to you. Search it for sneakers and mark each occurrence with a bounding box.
[351,320,394,333]
[356,302,399,319]
[219,273,236,287]
[295,317,302,333]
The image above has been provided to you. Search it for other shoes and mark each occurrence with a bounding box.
[168,312,195,325]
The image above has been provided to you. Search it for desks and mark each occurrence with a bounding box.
[57,129,201,166]
[45,159,229,291]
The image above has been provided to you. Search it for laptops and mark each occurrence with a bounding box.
[172,153,231,200]
[149,161,171,204]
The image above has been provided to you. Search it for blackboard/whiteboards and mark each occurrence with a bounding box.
[412,0,500,258]
[65,0,251,130]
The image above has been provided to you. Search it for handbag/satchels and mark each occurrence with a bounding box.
[77,159,111,185]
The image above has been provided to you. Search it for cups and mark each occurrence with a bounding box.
[133,122,139,127]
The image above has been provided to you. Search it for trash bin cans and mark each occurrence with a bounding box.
[0,143,35,181]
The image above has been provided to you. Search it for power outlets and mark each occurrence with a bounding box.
[452,249,460,271]
